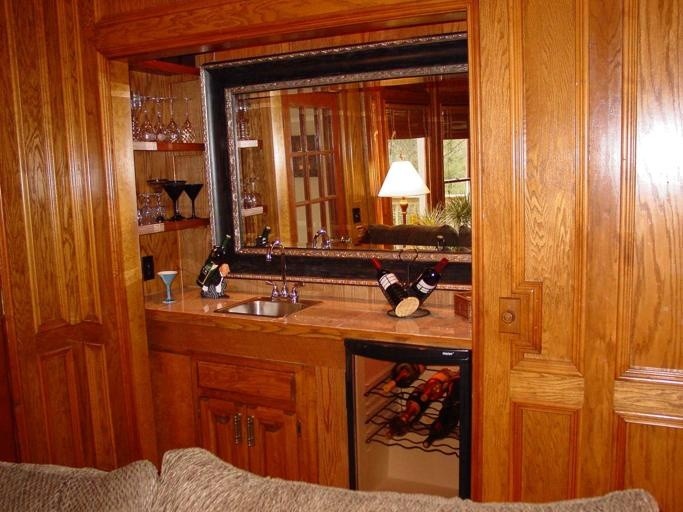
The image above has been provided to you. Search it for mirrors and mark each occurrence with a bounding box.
[199,31,474,292]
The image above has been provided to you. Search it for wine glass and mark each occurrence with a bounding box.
[135,192,165,226]
[156,270,178,304]
[235,99,250,139]
[145,178,168,222]
[162,180,185,220]
[184,183,202,219]
[238,177,260,209]
[130,93,195,143]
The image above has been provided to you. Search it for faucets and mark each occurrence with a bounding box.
[265,239,289,297]
[311,229,331,249]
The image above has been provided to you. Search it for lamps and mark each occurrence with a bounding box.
[377,160,430,225]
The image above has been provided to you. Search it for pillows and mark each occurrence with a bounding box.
[362,223,472,245]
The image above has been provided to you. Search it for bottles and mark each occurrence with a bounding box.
[254,226,271,248]
[369,255,410,311]
[196,234,232,287]
[410,257,449,305]
[379,361,460,448]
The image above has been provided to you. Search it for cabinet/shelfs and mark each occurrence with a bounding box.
[233,94,272,244]
[150,310,349,489]
[127,56,210,235]
[468,0,683,512]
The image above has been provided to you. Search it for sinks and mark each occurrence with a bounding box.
[213,295,323,319]
